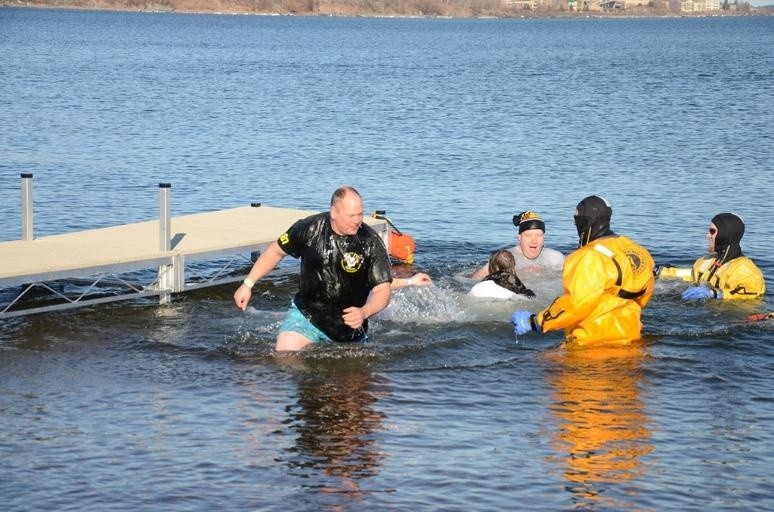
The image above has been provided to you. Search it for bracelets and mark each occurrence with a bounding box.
[407,278,412,285]
[244,278,255,288]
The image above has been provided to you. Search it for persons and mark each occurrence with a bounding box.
[653,212,767,303]
[280,359,389,511]
[466,249,536,300]
[543,350,654,511]
[234,186,392,352]
[390,272,433,289]
[509,194,657,346]
[471,211,566,278]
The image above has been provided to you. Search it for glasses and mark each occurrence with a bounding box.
[709,228,716,234]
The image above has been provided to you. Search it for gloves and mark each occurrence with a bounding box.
[512,310,533,335]
[682,286,723,300]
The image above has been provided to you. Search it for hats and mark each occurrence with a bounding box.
[711,212,745,262]
[513,211,546,233]
[574,196,612,244]
